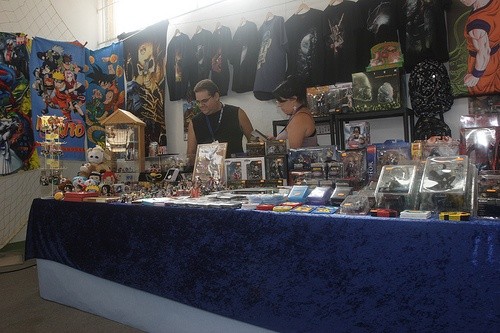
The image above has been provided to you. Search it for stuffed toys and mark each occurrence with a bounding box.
[58,146,116,197]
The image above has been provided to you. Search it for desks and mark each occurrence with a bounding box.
[24,196,500,333]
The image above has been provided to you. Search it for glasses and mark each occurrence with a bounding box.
[195,97,212,105]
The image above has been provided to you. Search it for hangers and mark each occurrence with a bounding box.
[263,11,275,22]
[294,3,311,16]
[238,17,248,27]
[328,0,344,6]
[214,22,224,31]
[173,28,183,37]
[196,25,204,34]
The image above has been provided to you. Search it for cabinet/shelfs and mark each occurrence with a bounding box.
[271,106,415,151]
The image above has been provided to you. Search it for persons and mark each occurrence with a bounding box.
[179,79,255,169]
[267,75,318,149]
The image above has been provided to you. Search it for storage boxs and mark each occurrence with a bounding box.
[143,112,500,222]
[63,191,121,204]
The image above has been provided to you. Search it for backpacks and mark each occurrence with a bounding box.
[410,57,454,122]
[414,117,452,140]
[398,0,450,71]
